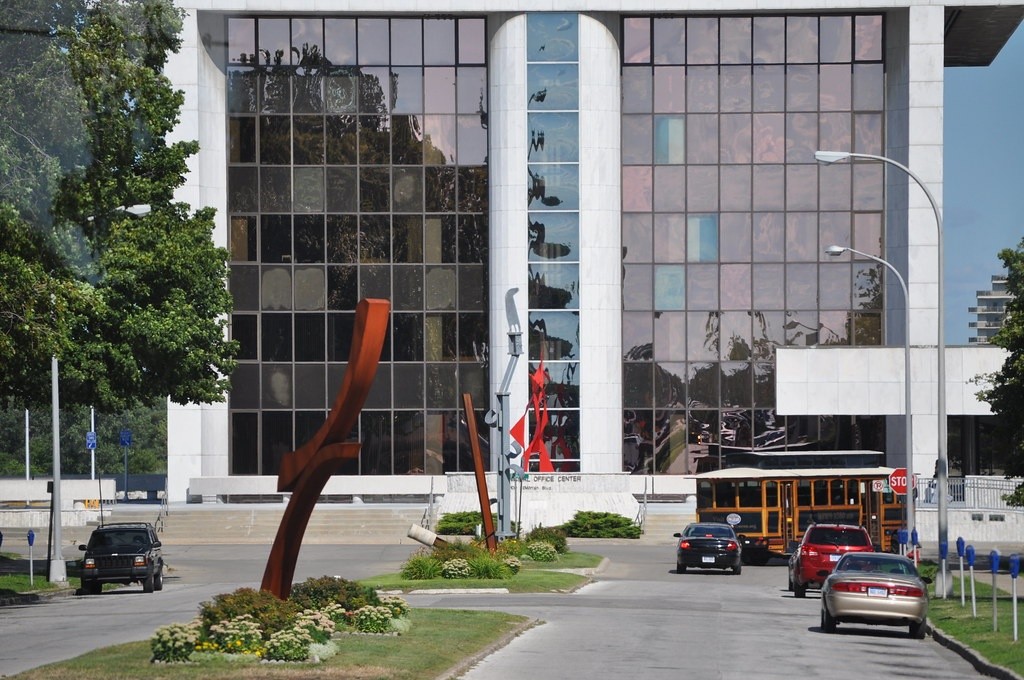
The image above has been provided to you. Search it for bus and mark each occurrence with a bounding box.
[690,468,919,567]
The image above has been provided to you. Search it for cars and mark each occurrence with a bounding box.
[673,522,745,577]
[786,547,821,591]
[819,551,934,638]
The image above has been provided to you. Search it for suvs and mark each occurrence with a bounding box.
[792,521,876,596]
[77,522,164,593]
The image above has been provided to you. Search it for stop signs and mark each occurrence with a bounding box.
[888,469,915,495]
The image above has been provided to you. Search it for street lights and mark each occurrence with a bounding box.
[824,243,917,558]
[813,150,951,601]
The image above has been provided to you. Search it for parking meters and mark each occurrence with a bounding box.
[939,540,949,600]
[988,549,1002,631]
[1007,553,1021,641]
[897,526,918,570]
[955,537,965,607]
[965,545,976,617]
[27,529,35,587]
